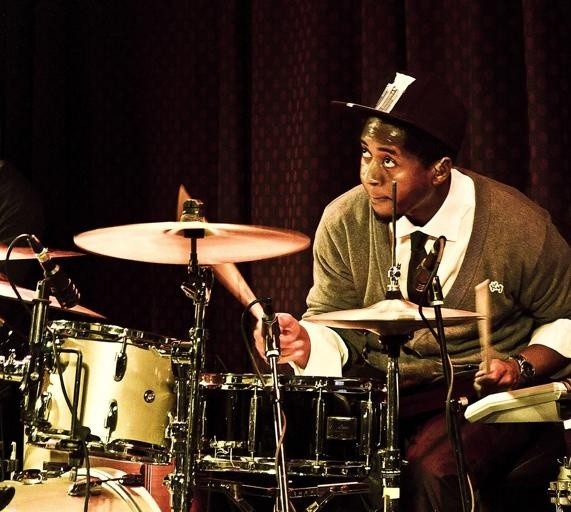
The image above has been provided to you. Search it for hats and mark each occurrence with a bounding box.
[330,72,470,153]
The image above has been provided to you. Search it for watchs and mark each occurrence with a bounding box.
[508,354,537,386]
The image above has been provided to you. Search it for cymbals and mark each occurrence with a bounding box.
[1,274,105,321]
[73,221,311,266]
[1,243,85,262]
[305,299,487,336]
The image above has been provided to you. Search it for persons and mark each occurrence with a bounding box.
[0,156,47,396]
[252,71,571,512]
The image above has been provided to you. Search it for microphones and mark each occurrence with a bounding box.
[26,233,81,309]
[261,298,282,363]
[410,235,448,295]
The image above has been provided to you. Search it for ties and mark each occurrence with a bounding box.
[407,231,434,307]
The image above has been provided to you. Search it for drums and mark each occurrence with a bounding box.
[0,350,33,483]
[26,320,177,465]
[195,373,388,512]
[1,467,162,512]
